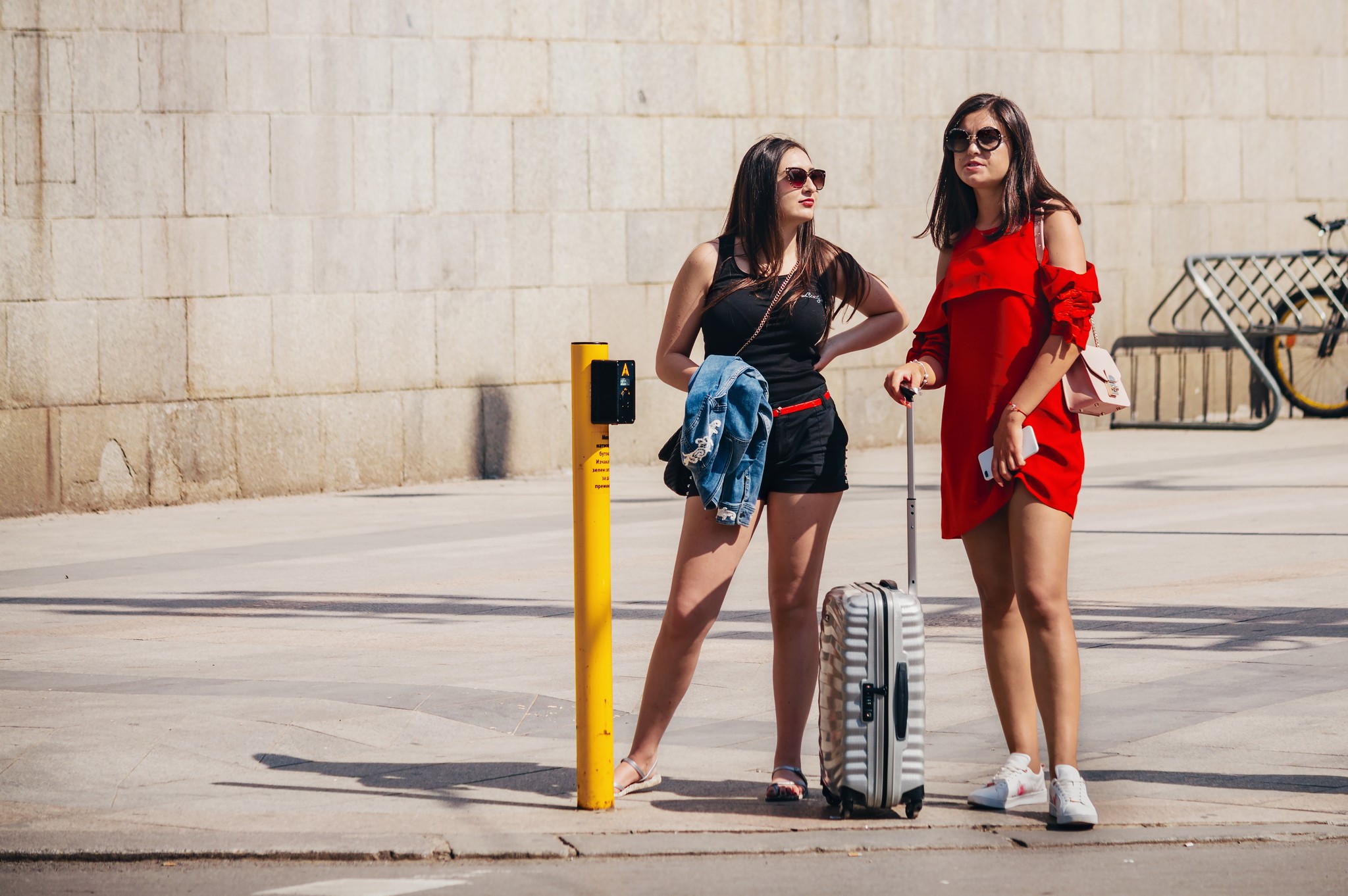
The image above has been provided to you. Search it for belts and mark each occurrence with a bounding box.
[772,390,831,417]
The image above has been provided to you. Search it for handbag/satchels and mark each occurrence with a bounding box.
[1062,345,1130,417]
[657,426,692,495]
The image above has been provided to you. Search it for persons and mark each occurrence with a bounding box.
[884,95,1101,824]
[609,138,910,802]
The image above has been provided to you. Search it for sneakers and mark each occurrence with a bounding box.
[1048,763,1098,825]
[967,753,1048,810]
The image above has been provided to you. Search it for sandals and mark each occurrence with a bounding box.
[614,757,661,797]
[765,766,808,799]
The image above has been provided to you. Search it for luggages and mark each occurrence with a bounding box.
[817,385,927,818]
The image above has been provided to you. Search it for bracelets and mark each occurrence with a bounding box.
[1008,401,1028,417]
[909,359,928,396]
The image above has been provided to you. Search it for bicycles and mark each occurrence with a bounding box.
[1262,212,1348,420]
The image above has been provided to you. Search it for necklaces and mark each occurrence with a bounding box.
[992,220,1000,227]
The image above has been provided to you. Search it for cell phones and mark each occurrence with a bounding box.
[978,426,1039,481]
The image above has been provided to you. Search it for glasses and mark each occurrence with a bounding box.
[778,166,826,190]
[946,126,1014,153]
